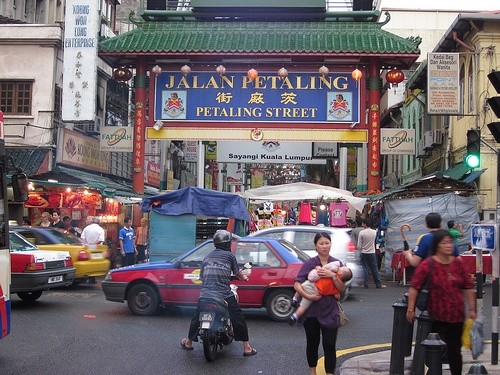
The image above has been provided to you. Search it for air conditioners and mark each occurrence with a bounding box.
[416,128,443,157]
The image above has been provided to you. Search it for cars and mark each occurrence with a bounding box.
[460,247,491,257]
[7,220,111,290]
[9,231,76,303]
[237,225,364,287]
[101,237,355,324]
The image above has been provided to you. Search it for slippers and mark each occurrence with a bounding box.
[181,339,193,350]
[243,347,257,356]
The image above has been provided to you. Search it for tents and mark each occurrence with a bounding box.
[235,182,368,228]
[142,185,251,263]
[362,172,480,278]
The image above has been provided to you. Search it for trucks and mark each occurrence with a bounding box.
[257,202,273,219]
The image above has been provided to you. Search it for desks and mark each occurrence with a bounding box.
[391,251,492,286]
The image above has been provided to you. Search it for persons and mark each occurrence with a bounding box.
[294,231,345,375]
[289,265,353,326]
[22,219,31,226]
[40,211,105,284]
[403,212,488,375]
[118,217,138,268]
[181,229,256,353]
[350,216,364,244]
[134,217,148,264]
[351,222,356,228]
[357,218,387,288]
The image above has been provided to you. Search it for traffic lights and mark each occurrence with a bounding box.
[466,129,480,167]
[486,68,500,144]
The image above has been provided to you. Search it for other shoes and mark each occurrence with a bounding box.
[289,314,298,324]
[291,300,299,308]
[380,285,386,288]
[361,285,366,288]
[88,281,97,283]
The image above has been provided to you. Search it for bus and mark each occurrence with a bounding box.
[0,110,11,341]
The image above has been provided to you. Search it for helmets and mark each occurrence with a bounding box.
[213,229,232,247]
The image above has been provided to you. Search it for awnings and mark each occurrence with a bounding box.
[5,166,159,199]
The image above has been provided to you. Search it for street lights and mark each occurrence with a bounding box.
[320,204,325,224]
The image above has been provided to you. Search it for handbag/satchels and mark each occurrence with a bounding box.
[415,261,434,311]
[469,320,484,360]
[462,318,474,350]
